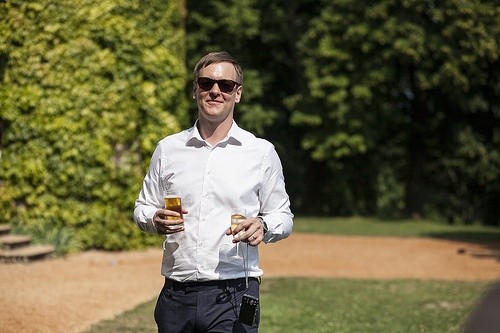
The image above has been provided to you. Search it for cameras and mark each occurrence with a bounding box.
[239,294,258,326]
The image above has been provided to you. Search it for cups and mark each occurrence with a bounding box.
[162,189,184,228]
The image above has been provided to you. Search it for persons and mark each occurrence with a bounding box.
[132,53,294,332]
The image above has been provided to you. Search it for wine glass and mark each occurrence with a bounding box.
[229,208,247,261]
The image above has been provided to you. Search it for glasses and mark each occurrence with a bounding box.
[194,76,242,92]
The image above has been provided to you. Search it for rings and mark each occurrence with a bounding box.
[252,234,256,239]
